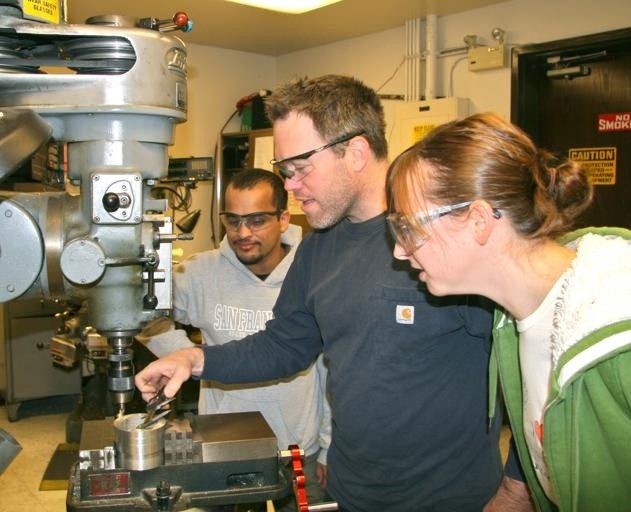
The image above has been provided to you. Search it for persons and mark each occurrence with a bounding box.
[133,73,538,511]
[131,166,330,512]
[382,110,630,511]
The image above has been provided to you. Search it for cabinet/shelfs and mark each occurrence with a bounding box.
[215,127,314,241]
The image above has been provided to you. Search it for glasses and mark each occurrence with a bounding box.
[384,197,502,255]
[218,210,281,233]
[268,128,367,183]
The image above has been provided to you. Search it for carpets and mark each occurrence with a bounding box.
[39,442,80,491]
